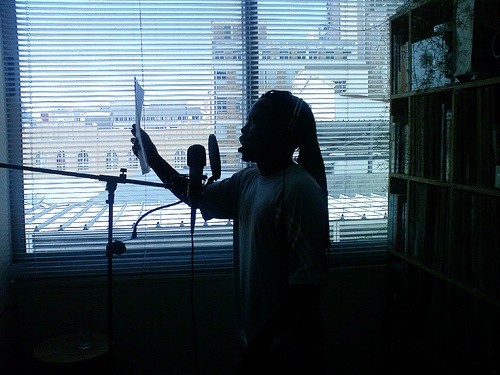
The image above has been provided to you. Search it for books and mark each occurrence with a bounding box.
[389,32,500,296]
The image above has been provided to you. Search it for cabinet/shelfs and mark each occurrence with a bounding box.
[383,1,499,374]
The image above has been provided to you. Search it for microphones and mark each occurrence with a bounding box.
[186,144,206,209]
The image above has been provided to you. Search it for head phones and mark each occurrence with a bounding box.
[246,99,302,161]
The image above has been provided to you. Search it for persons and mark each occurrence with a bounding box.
[131,90,330,375]
